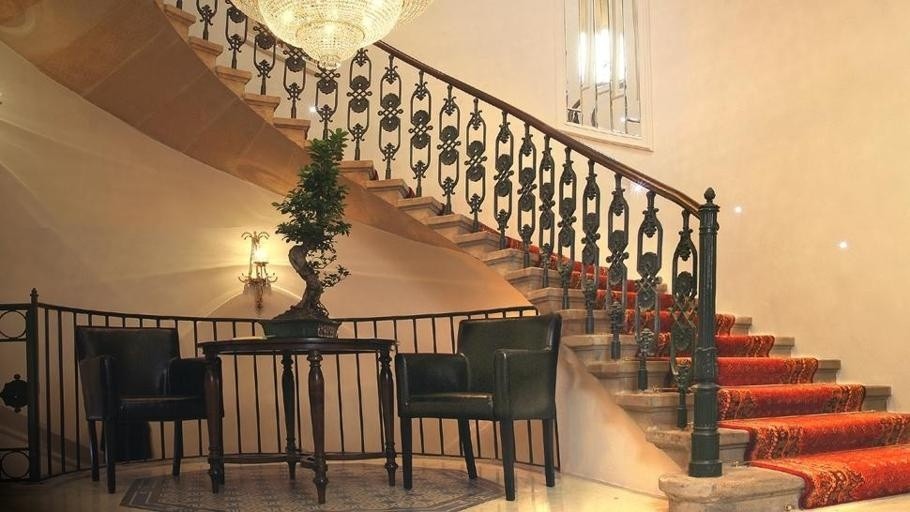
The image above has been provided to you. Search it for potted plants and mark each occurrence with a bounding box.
[252,127,344,339]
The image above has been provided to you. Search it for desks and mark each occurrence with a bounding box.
[196,339,401,501]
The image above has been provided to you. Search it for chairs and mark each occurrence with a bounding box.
[70,314,562,502]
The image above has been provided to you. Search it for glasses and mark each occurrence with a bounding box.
[249,0,436,71]
[236,229,279,314]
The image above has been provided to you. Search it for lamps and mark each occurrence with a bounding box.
[249,0,436,71]
[236,229,279,314]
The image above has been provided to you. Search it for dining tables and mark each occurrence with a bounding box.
[252,127,344,339]
